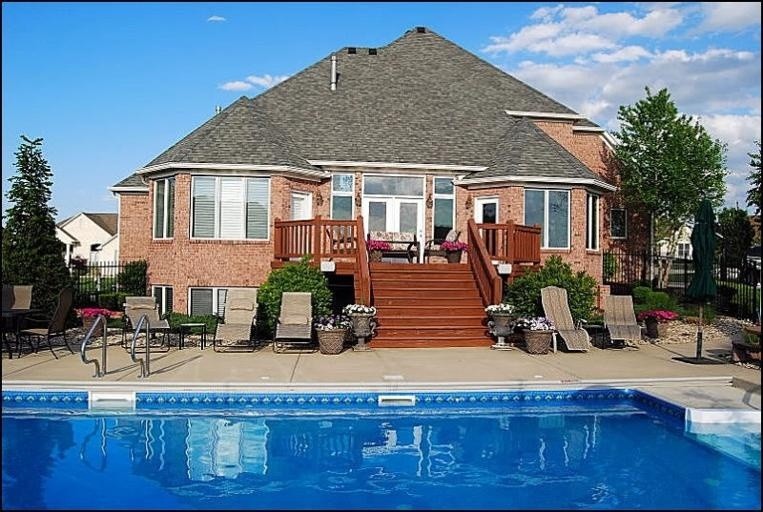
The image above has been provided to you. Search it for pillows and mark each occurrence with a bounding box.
[281,314,307,325]
[125,298,155,309]
[229,297,253,311]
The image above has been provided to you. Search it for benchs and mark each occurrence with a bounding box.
[365,230,420,263]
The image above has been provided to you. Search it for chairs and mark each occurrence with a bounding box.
[538,286,591,353]
[0,283,75,359]
[601,293,640,351]
[422,228,462,263]
[325,228,371,262]
[270,289,313,355]
[121,295,170,355]
[211,285,259,353]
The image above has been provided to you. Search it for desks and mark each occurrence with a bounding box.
[178,322,206,351]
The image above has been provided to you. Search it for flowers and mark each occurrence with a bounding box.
[342,303,376,313]
[482,303,517,316]
[315,315,351,331]
[364,239,390,252]
[76,306,110,319]
[637,309,678,322]
[439,240,468,252]
[517,315,556,332]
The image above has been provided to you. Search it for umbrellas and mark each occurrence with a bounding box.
[684,198,719,358]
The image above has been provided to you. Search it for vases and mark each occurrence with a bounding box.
[520,328,553,354]
[81,317,103,337]
[445,250,462,263]
[315,328,345,355]
[367,250,383,263]
[645,317,669,338]
[486,313,515,350]
[348,312,373,349]
